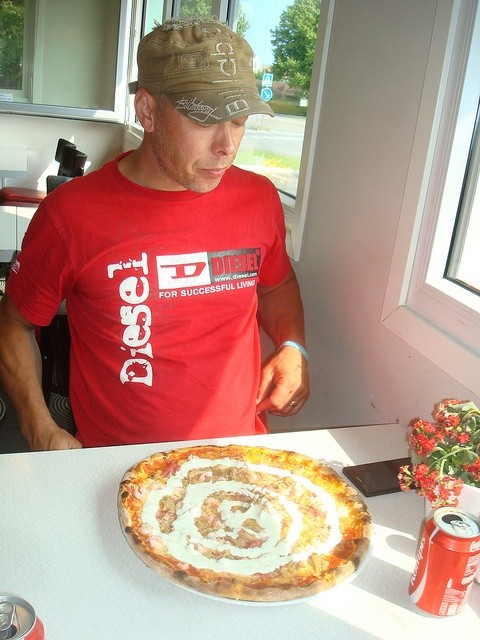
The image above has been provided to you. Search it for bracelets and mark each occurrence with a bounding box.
[274,340,309,361]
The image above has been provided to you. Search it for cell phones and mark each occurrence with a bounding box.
[341,456,423,497]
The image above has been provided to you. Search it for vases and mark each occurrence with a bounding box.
[423,478,480,585]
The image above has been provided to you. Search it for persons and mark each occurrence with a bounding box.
[3,20,316,451]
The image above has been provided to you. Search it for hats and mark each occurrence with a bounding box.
[128,17,275,124]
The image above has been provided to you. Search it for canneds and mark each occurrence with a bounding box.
[0,592,47,640]
[408,507,480,621]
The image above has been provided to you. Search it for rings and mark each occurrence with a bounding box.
[290,400,299,408]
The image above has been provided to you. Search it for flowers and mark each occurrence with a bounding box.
[398,400,480,510]
